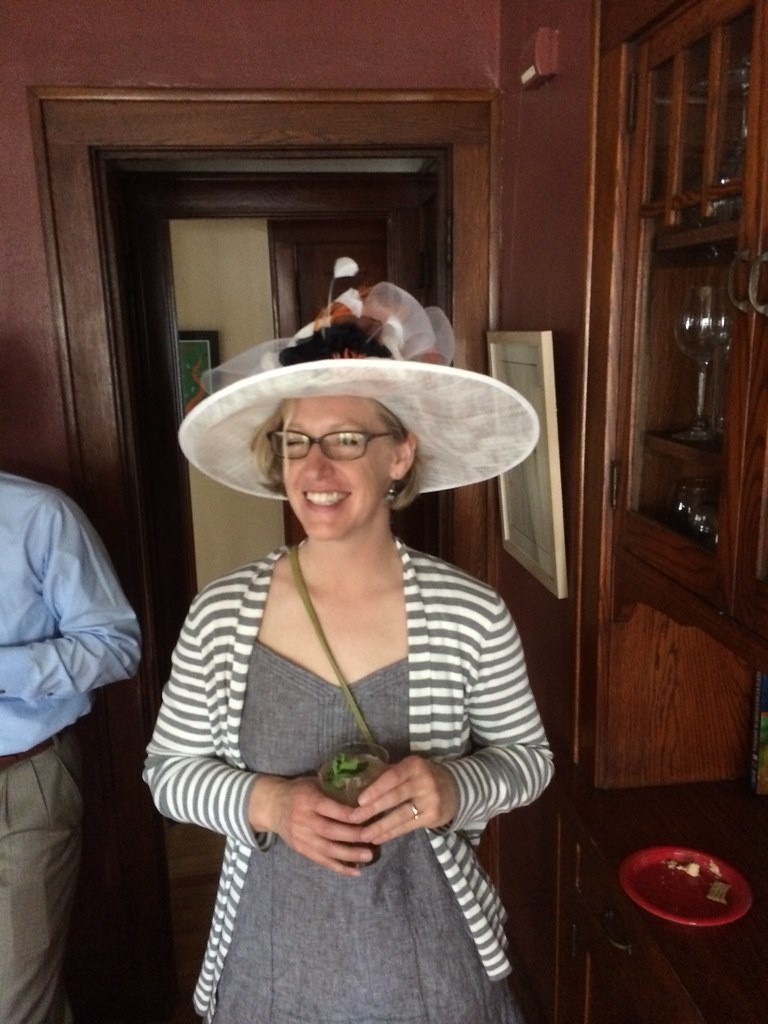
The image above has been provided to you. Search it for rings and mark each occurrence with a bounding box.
[411,802,418,815]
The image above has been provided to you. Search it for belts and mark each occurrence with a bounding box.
[0,725,68,774]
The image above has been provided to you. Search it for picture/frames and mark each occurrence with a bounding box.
[485,328,571,601]
[177,330,220,424]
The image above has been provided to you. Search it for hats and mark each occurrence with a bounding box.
[177,282,540,500]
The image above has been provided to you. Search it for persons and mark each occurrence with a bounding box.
[0,472,139,1024]
[140,360,555,1024]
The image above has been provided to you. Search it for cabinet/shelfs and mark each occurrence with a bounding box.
[607,0,768,673]
[560,776,768,1024]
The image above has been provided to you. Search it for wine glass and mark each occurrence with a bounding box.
[673,284,734,444]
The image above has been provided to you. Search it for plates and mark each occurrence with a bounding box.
[618,844,753,927]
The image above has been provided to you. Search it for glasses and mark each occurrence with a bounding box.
[266,421,397,462]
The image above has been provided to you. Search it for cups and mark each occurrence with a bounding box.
[317,742,389,868]
[674,482,717,535]
